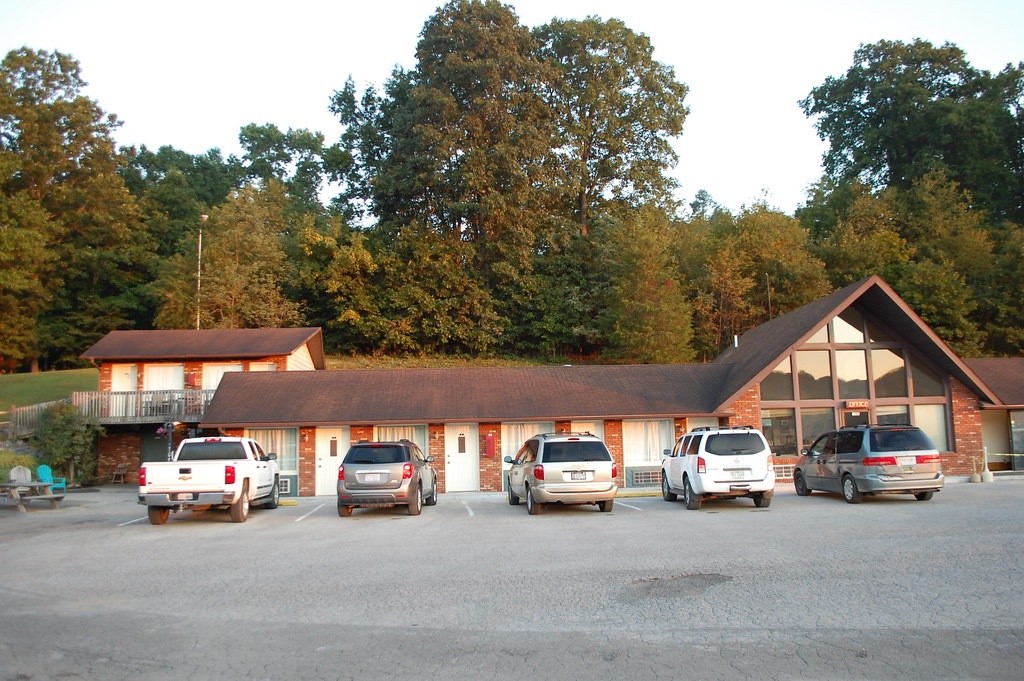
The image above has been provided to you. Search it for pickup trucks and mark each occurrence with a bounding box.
[137,435,280,524]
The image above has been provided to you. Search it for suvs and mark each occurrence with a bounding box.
[336,439,437,518]
[792,422,945,504]
[661,425,775,510]
[501,430,619,515]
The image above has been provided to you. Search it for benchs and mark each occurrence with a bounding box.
[104,464,129,485]
[23,494,65,510]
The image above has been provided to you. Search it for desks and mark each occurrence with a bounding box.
[0,481,60,513]
[172,396,193,415]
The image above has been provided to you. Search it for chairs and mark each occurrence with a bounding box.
[187,394,204,416]
[144,391,168,415]
[9,465,34,496]
[35,464,67,494]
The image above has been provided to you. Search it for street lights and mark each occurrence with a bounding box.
[196,214,209,329]
